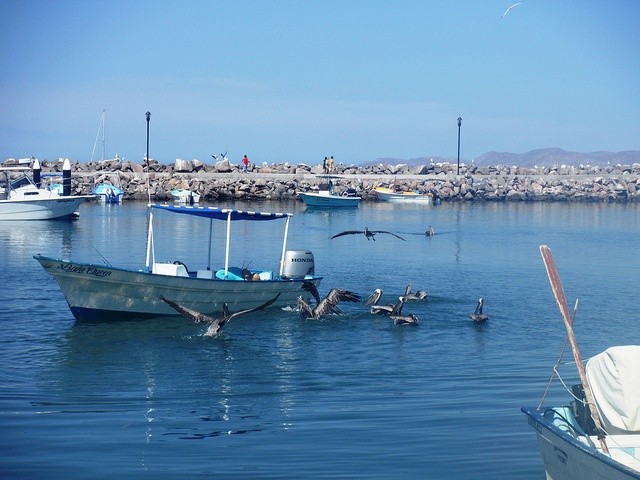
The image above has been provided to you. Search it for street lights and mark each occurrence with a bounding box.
[456,116,463,175]
[144,110,152,173]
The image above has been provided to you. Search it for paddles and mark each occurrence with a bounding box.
[540,243,608,454]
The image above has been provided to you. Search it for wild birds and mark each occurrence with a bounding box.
[301,281,361,315]
[296,296,343,322]
[404,282,430,304]
[329,226,406,243]
[363,287,396,316]
[393,224,455,238]
[386,295,420,327]
[469,298,489,326]
[157,290,281,340]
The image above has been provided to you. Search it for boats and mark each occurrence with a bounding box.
[295,190,362,206]
[91,183,124,204]
[520,297,640,480]
[373,186,434,203]
[32,202,324,323]
[0,166,98,219]
[169,189,200,203]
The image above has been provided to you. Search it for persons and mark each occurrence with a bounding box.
[323,157,329,174]
[242,155,249,171]
[329,156,335,173]
